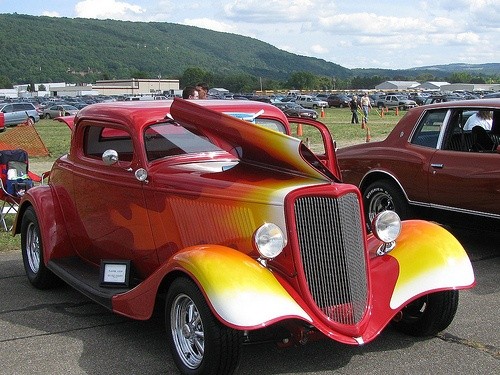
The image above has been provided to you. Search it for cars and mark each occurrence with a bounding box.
[12,99,478,374]
[210,91,500,118]
[319,98,500,239]
[0,95,182,119]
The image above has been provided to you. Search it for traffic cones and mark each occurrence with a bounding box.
[321,107,324,117]
[395,107,398,115]
[386,106,388,112]
[361,120,365,128]
[381,108,383,117]
[366,128,370,142]
[297,115,302,136]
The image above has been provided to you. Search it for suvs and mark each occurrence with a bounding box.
[0,102,40,127]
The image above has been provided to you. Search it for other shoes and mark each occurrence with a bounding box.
[365,121,367,123]
[356,122,360,124]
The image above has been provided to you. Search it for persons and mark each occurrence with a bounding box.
[183,83,209,100]
[349,92,371,124]
[463,111,492,131]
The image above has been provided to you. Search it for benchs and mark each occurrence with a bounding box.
[418,129,499,149]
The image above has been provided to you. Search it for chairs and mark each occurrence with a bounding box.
[0,148,51,231]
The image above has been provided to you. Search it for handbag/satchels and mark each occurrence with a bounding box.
[2,160,33,196]
